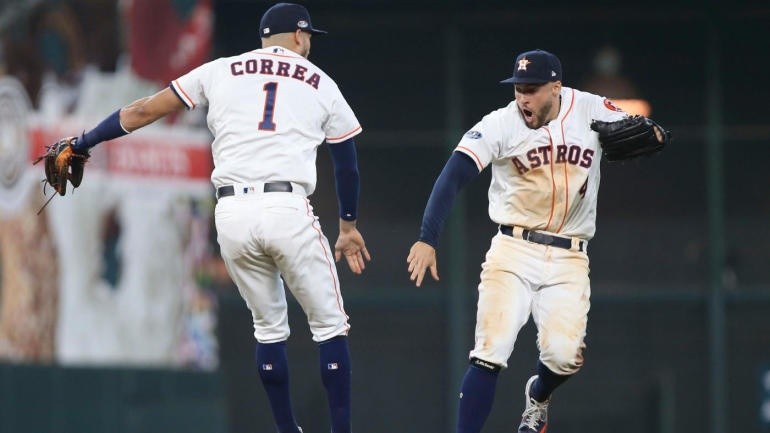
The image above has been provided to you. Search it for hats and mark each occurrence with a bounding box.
[258,2,329,38]
[499,48,562,84]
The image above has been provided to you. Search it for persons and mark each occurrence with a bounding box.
[45,2,372,433]
[406,51,665,432]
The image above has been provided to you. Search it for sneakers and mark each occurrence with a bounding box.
[517,374,553,433]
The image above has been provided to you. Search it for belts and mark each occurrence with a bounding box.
[217,181,292,198]
[500,225,588,252]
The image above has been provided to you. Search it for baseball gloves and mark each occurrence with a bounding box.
[28,135,91,216]
[587,113,677,164]
